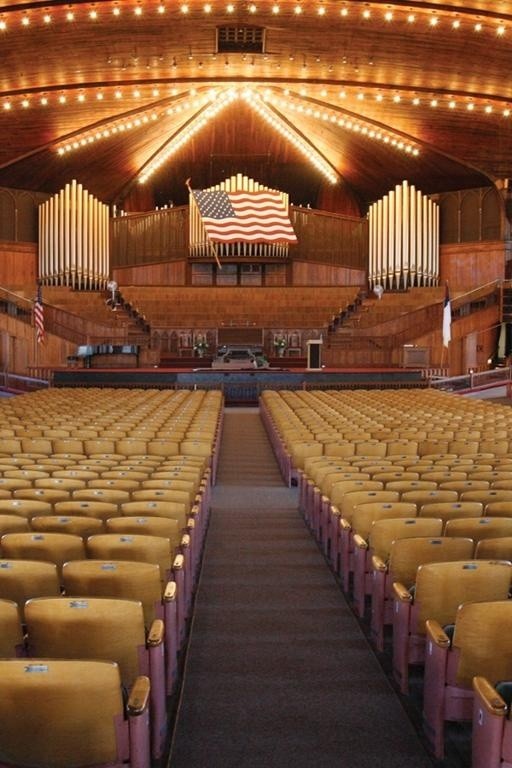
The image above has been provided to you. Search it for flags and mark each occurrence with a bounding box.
[189,190,300,245]
[35,286,46,344]
[441,280,453,347]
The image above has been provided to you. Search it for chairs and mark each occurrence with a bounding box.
[1,383,228,767]
[251,383,510,766]
